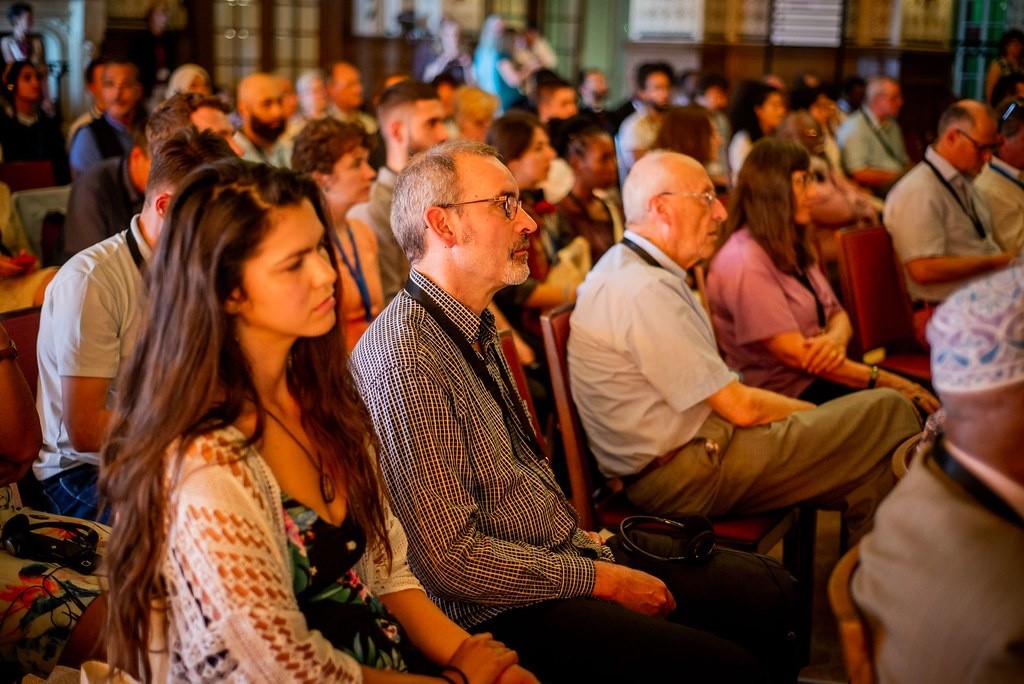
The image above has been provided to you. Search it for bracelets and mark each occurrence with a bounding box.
[90,106,101,120]
[439,664,469,684]
[868,365,878,388]
[0,340,19,360]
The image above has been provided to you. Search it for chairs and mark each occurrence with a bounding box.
[1,228,931,684]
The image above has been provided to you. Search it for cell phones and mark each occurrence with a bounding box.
[28,531,103,574]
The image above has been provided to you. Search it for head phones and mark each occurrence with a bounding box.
[618,512,717,568]
[1,513,98,564]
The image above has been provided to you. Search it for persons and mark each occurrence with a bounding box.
[0,0,1024,684]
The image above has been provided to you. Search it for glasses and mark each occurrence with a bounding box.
[424,195,522,230]
[648,190,718,211]
[957,128,1003,153]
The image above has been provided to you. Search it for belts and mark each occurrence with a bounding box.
[622,440,694,486]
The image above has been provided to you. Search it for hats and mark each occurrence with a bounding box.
[928,266,1024,394]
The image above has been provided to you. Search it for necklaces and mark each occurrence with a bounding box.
[922,155,986,239]
[126,228,152,290]
[989,163,1024,191]
[619,236,665,269]
[859,105,906,168]
[404,274,546,461]
[931,432,1024,533]
[331,222,374,322]
[246,389,336,503]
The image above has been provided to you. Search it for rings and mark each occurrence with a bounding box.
[915,396,921,401]
